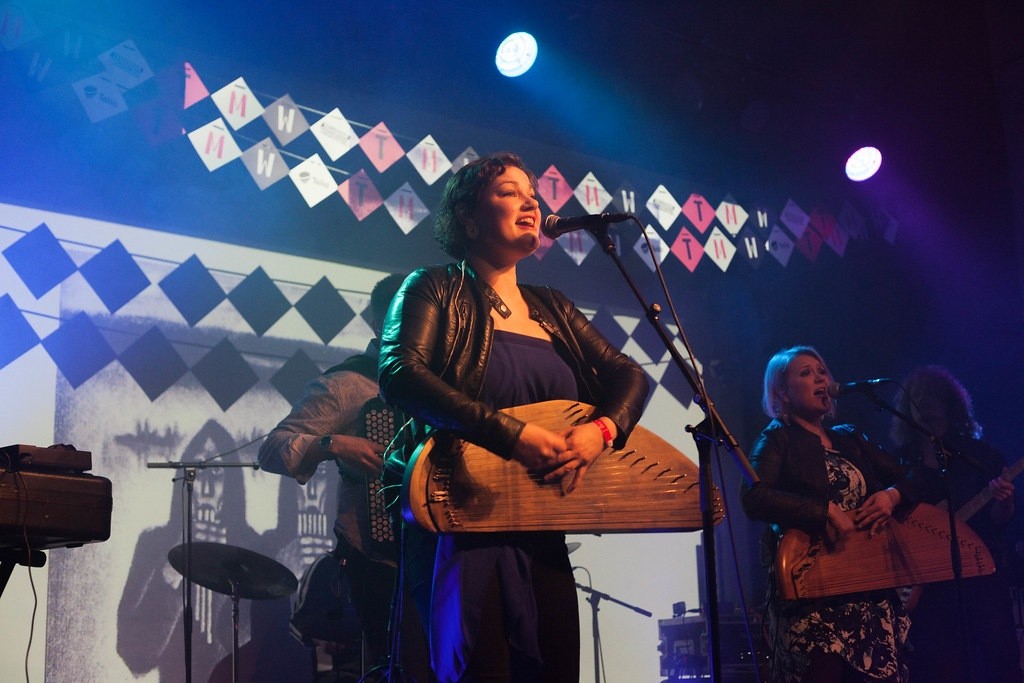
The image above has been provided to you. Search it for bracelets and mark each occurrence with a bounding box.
[593,418,614,450]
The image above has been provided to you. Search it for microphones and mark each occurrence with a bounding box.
[541,212,632,240]
[828,377,892,398]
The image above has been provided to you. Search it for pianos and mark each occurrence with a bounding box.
[0,470,113,551]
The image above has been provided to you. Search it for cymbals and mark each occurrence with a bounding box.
[566,542,581,554]
[167,539,300,601]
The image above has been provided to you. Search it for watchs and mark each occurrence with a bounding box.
[318,434,339,462]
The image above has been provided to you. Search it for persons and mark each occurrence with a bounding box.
[377,152,651,683]
[257,272,408,683]
[739,344,923,683]
[888,365,1016,683]
